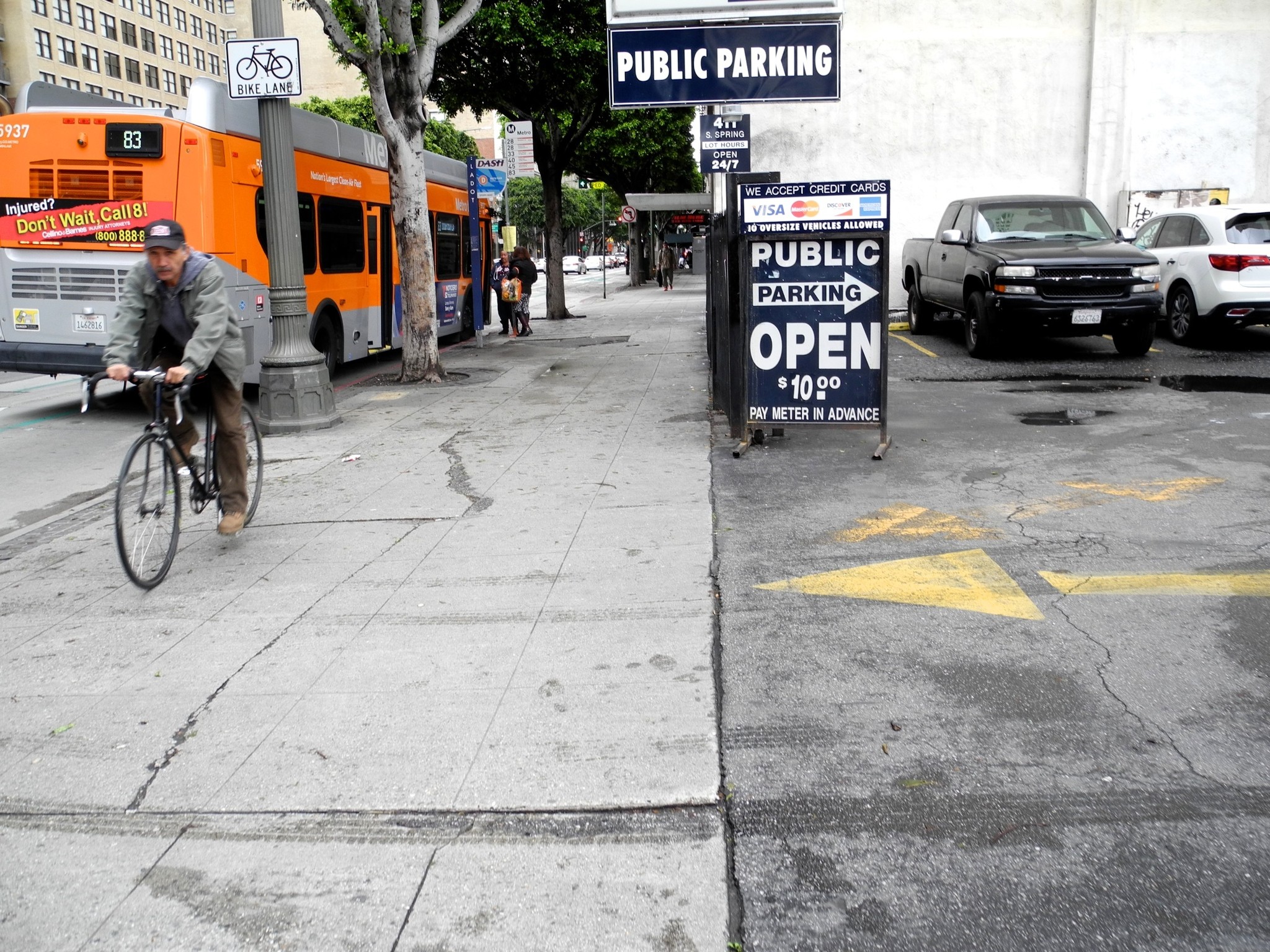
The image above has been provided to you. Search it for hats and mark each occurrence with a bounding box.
[663,241,668,243]
[145,219,184,250]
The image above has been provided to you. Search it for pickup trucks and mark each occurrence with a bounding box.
[903,193,1167,358]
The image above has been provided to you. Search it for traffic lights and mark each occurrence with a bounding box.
[580,238,584,243]
[578,248,581,252]
[578,176,588,189]
[618,215,622,222]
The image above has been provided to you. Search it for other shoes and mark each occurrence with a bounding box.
[669,283,673,290]
[664,287,667,290]
[498,330,509,335]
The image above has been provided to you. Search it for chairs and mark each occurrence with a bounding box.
[985,218,994,233]
[1170,220,1201,246]
[1024,223,1043,232]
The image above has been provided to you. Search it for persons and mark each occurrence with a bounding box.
[102,219,249,535]
[679,251,692,269]
[490,246,538,337]
[656,241,677,291]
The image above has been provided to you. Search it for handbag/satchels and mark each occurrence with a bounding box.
[500,277,520,301]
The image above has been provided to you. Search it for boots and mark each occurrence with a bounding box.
[516,311,533,336]
[509,327,519,337]
[518,313,530,336]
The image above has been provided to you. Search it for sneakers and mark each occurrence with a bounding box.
[218,510,246,533]
[166,429,199,468]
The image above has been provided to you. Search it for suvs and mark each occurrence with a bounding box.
[1122,204,1270,343]
[614,253,627,267]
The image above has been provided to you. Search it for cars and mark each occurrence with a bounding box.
[599,256,614,269]
[531,258,547,274]
[561,256,586,275]
[584,256,603,271]
[609,256,620,268]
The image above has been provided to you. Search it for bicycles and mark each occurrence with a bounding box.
[74,368,267,589]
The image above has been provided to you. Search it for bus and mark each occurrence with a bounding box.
[1,76,507,388]
